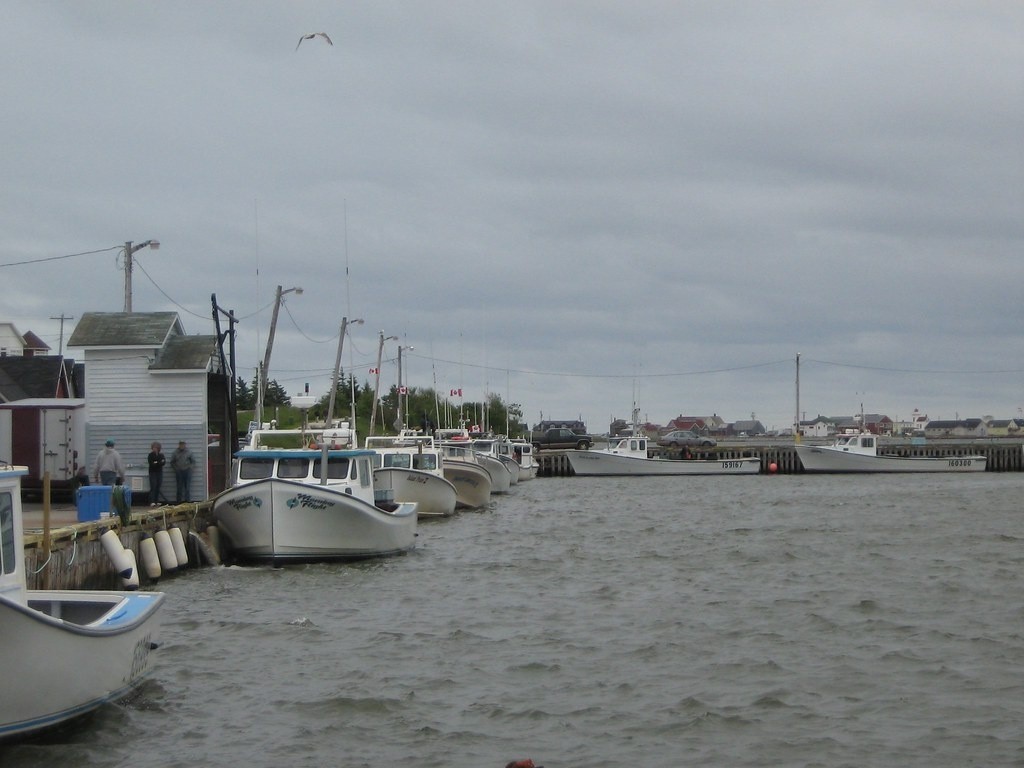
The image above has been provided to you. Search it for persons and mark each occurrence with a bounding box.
[95,438,125,486]
[170,440,195,505]
[148,443,166,507]
[422,414,435,434]
[682,445,693,460]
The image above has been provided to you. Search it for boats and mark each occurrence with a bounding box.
[210,382,420,568]
[365,400,540,520]
[0,462,164,747]
[534,377,762,478]
[794,404,988,473]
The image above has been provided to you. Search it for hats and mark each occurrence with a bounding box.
[179,440,186,444]
[105,439,116,446]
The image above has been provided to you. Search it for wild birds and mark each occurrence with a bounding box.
[294,31,332,52]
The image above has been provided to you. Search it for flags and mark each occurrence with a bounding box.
[470,424,481,433]
[450,389,462,396]
[369,368,378,374]
[397,386,407,395]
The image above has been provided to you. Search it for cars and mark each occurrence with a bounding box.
[755,432,766,437]
[656,430,718,449]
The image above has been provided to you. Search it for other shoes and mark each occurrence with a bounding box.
[151,503,155,507]
[157,503,162,505]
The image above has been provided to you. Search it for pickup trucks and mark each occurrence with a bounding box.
[522,427,595,455]
